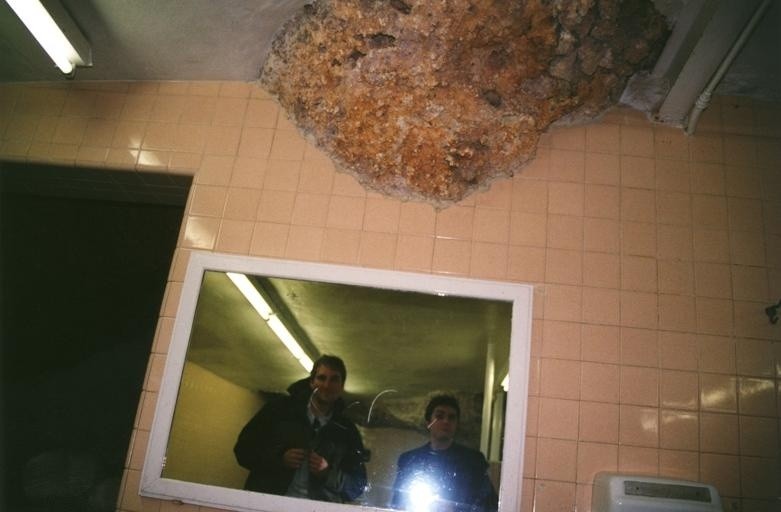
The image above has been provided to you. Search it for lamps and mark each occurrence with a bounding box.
[2,0,96,85]
[267,311,318,374]
[223,270,277,320]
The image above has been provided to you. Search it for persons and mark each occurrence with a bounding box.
[390,392,500,512]
[231,352,372,505]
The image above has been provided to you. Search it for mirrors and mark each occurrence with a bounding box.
[137,247,537,512]
